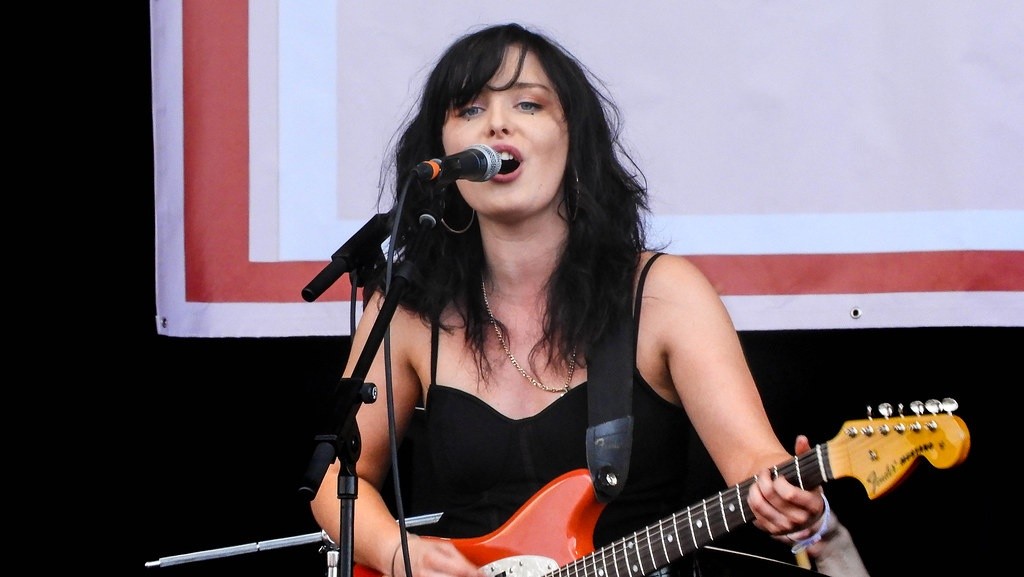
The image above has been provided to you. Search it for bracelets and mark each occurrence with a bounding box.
[787,492,830,554]
[389,533,417,577]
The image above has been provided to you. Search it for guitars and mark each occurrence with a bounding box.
[354,394,973,577]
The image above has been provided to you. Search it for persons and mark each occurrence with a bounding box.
[311,24,828,577]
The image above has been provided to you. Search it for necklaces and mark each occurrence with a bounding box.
[475,275,579,397]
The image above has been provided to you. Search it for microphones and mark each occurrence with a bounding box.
[410,144,503,182]
